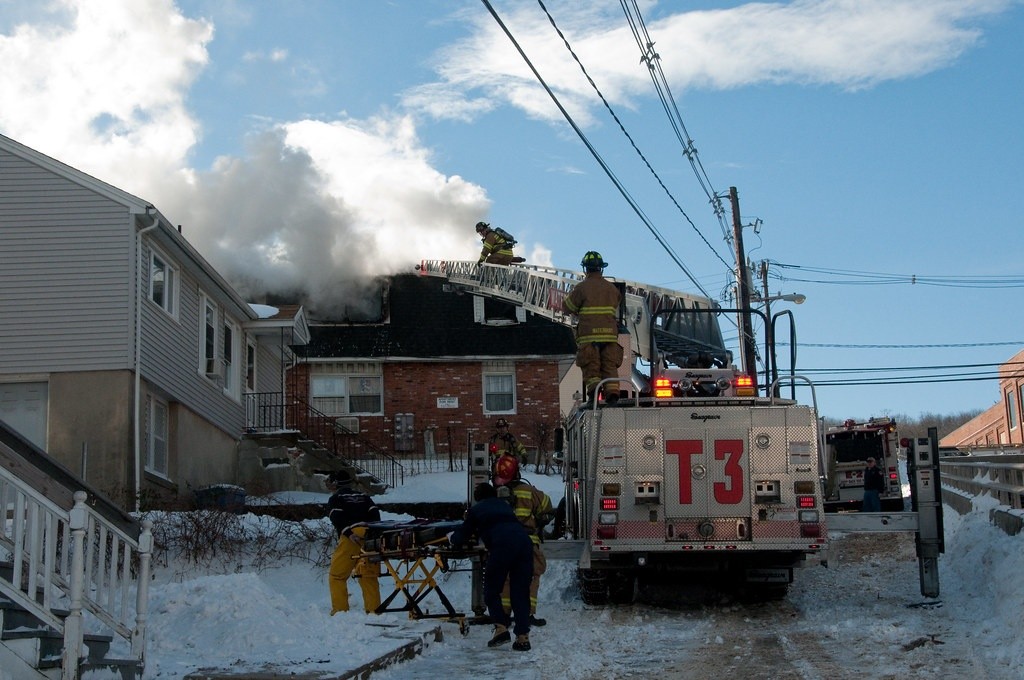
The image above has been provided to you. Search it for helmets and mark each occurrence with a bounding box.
[580,251,608,276]
[475,221,490,239]
[495,418,509,428]
[492,453,519,486]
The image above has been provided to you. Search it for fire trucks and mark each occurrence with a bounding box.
[818,417,905,513]
[415,260,942,606]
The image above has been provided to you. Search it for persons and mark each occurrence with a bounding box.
[475,221,513,284]
[562,251,624,410]
[323,466,383,616]
[446,482,534,651]
[492,455,554,626]
[489,416,529,467]
[862,457,885,512]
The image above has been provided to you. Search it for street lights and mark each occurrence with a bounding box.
[736,294,806,397]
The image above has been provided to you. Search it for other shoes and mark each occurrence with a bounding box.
[528,614,546,626]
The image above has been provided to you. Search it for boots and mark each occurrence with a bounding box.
[606,393,619,406]
[579,388,602,410]
[487,623,512,647]
[512,632,531,651]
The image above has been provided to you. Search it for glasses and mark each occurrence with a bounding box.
[867,461,872,463]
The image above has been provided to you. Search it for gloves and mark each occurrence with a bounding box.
[522,456,528,467]
[478,259,484,267]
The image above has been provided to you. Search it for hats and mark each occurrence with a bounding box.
[866,457,876,462]
